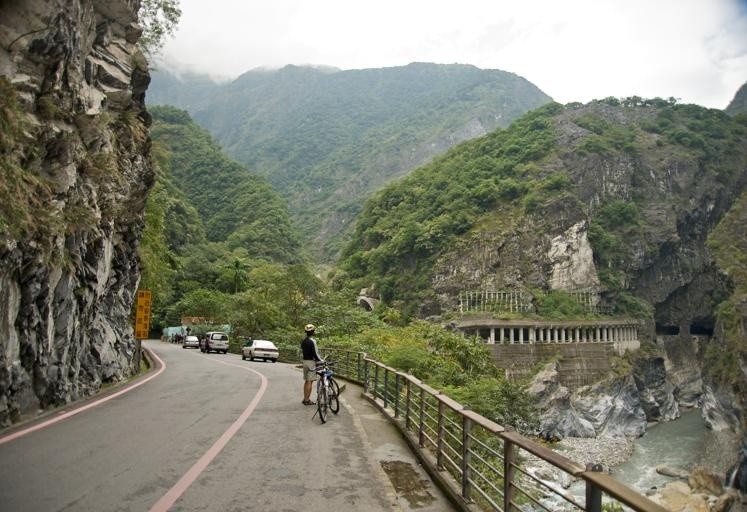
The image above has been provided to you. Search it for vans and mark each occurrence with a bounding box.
[200,331,230,354]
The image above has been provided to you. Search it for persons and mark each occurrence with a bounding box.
[300,323,323,405]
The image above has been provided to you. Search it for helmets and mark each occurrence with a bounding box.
[304,324,315,331]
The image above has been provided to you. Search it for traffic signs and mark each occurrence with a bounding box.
[134,289,151,340]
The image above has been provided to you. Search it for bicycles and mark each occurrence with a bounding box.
[309,352,340,400]
[311,361,341,425]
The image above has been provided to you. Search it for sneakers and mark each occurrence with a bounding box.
[302,399,317,405]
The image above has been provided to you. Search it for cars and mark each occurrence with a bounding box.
[182,335,199,349]
[240,339,280,363]
